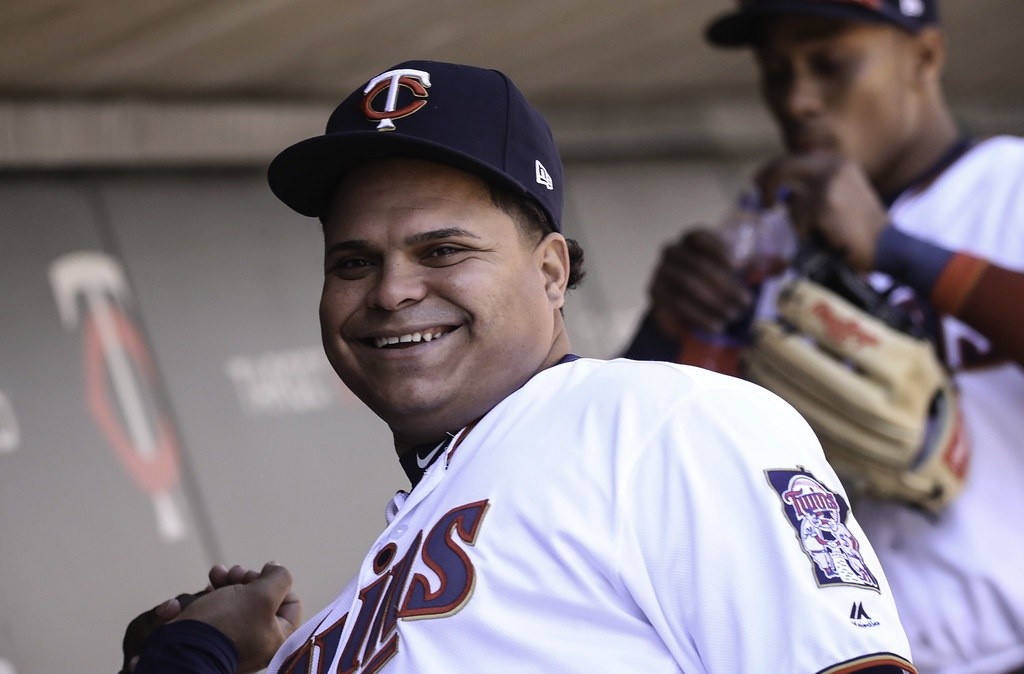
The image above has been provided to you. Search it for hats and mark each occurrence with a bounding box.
[705,0,939,50]
[268,61,561,231]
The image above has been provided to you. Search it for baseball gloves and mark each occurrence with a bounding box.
[735,247,972,522]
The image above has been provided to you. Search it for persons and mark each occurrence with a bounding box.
[119,561,303,674]
[618,0,1024,674]
[267,61,918,674]
